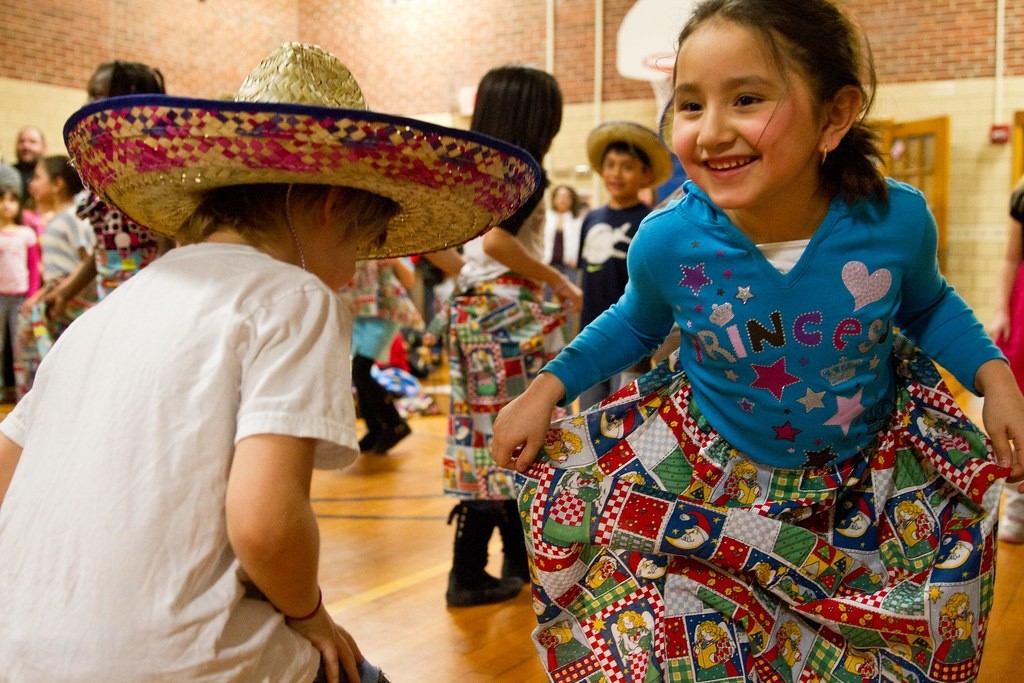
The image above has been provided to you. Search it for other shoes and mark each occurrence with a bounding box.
[358,429,379,451]
[997,504,1024,545]
[446,567,524,607]
[500,556,530,584]
[374,419,411,452]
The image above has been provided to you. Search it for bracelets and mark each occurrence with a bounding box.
[284,584,322,622]
[552,277,570,296]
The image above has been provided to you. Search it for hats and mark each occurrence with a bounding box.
[63,41,542,260]
[583,117,674,191]
[658,94,674,153]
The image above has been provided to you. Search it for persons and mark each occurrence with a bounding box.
[0,40,543,683]
[490,0,1024,683]
[575,120,674,414]
[0,61,464,458]
[423,65,583,607]
[984,175,1024,545]
[543,184,584,347]
